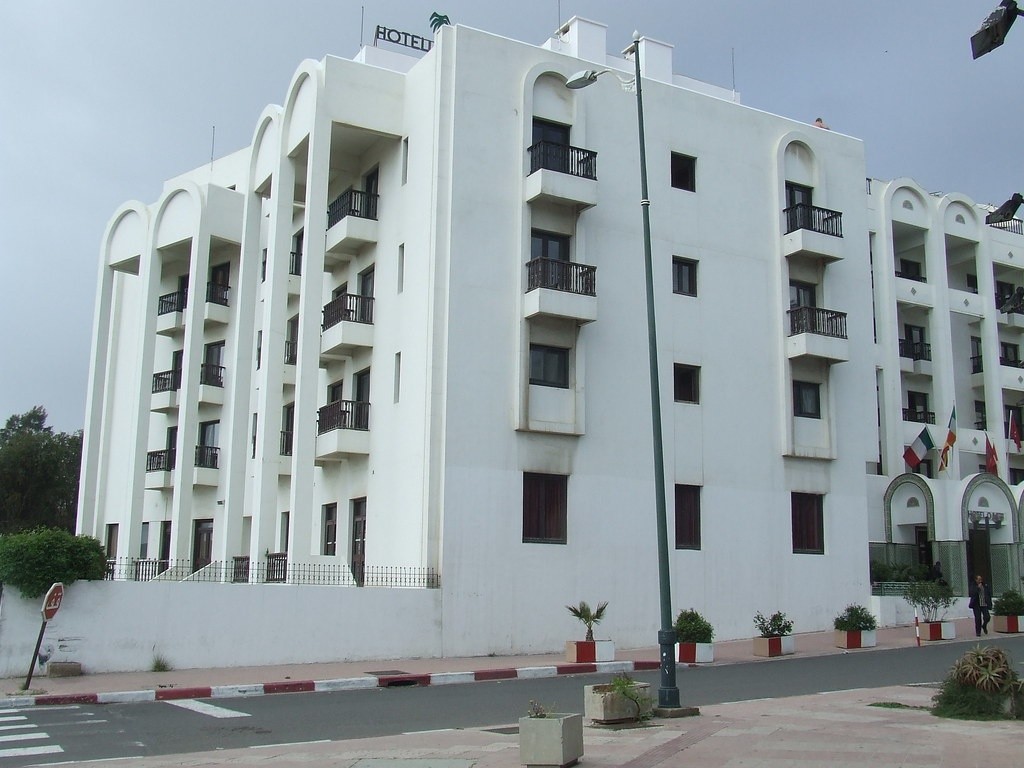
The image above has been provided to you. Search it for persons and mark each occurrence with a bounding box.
[968,574,993,637]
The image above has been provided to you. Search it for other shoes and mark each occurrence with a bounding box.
[983,625,987,634]
[976,633,981,637]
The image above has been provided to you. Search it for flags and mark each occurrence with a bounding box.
[986,437,997,476]
[937,405,956,470]
[1010,412,1021,454]
[901,426,935,468]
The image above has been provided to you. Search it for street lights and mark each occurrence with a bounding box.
[566,29,685,710]
